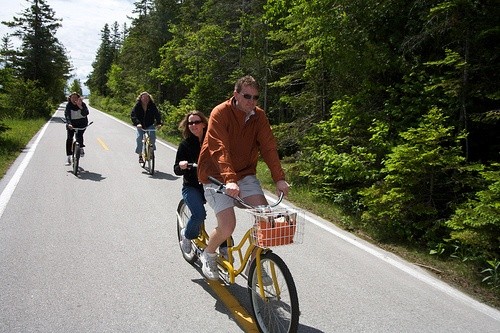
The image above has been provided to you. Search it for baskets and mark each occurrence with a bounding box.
[246,207,305,248]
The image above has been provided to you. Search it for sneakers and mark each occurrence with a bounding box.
[201,248,219,280]
[245,260,271,285]
[180,227,191,254]
[219,247,235,262]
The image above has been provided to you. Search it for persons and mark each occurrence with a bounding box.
[65,91,89,166]
[198,75,290,287]
[174,110,235,262]
[131,92,162,163]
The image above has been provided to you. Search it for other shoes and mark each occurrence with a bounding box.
[149,150,154,159]
[65,157,71,166]
[80,149,85,157]
[139,156,143,163]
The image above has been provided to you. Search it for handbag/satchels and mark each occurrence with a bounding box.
[256,215,297,246]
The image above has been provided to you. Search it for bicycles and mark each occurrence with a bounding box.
[173,162,307,332]
[139,127,161,175]
[61,115,94,176]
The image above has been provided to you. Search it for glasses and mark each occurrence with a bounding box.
[188,120,202,125]
[237,91,260,101]
